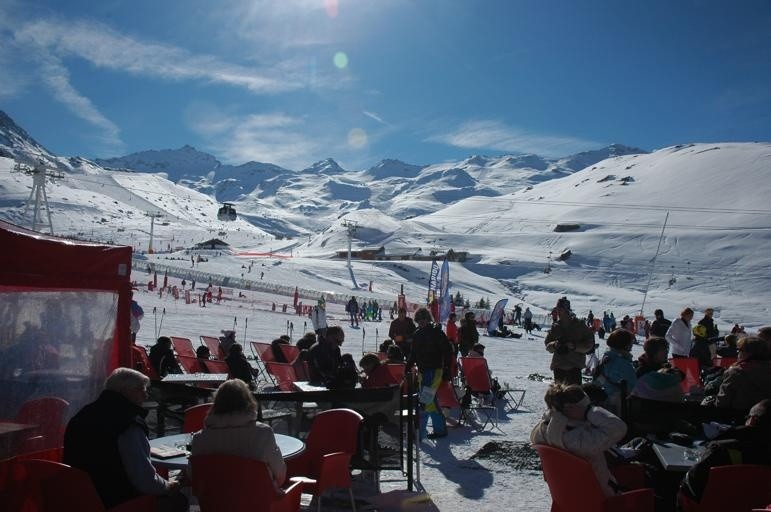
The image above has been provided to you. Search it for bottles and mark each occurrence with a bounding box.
[186,431,196,457]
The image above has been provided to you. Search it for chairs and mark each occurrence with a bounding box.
[593,336,739,435]
[676,463,770,511]
[532,442,655,512]
[1,396,106,511]
[280,407,363,511]
[129,333,526,436]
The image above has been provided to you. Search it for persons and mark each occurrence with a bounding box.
[528,298,770,511]
[129,292,500,468]
[496,304,533,339]
[63,367,188,511]
[189,378,288,496]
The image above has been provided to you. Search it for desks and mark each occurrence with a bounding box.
[148,432,305,468]
[651,437,699,471]
